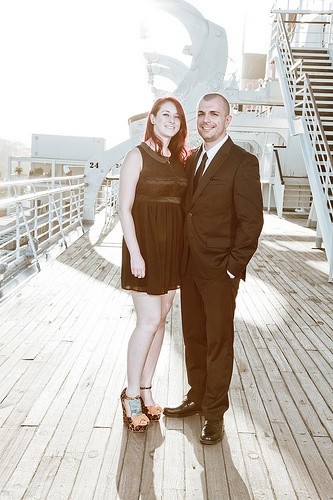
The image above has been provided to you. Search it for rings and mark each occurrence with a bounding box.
[138,274,140,276]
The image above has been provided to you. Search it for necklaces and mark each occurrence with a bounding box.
[147,138,173,166]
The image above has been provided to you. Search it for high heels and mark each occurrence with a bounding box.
[121,387,148,432]
[139,387,162,421]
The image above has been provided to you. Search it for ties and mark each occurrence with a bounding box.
[193,152,208,197]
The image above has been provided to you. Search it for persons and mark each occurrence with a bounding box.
[162,93,264,445]
[118,97,188,433]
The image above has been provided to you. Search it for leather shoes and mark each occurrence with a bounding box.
[199,418,224,445]
[163,398,205,418]
